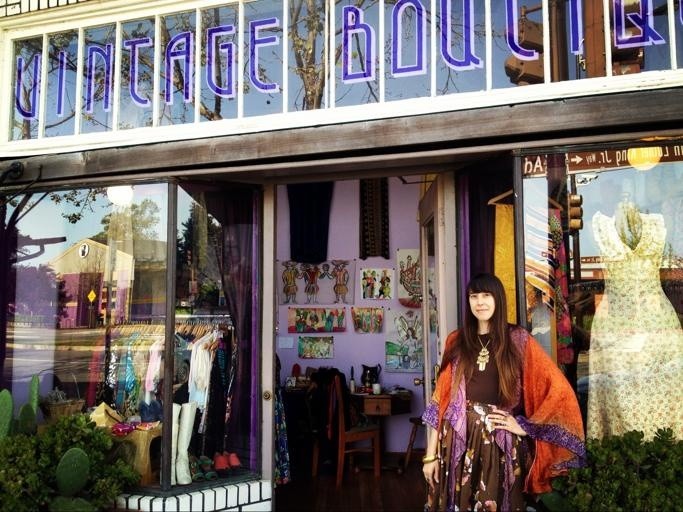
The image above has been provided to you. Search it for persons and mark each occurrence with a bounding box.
[421,271,584,512]
[400,329,422,370]
[585,191,681,446]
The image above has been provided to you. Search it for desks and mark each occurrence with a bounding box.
[360,393,412,471]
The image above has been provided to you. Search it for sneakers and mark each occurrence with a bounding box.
[213,452,232,478]
[223,450,245,477]
[139,401,156,422]
[150,400,164,421]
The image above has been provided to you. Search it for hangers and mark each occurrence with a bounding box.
[485,188,565,213]
[87,315,238,352]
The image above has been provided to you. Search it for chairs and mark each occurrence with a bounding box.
[308,373,381,494]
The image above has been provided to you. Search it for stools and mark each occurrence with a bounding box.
[401,416,425,473]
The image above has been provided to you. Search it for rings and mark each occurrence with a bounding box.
[426,477,430,482]
[503,411,508,416]
[502,419,505,424]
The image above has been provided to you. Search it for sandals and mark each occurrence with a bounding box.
[189,455,205,482]
[199,456,218,481]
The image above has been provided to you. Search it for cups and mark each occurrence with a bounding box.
[371,383,381,396]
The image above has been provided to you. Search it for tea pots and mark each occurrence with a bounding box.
[360,364,382,385]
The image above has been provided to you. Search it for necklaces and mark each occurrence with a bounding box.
[475,333,491,373]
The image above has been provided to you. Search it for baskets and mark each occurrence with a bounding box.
[36,368,86,422]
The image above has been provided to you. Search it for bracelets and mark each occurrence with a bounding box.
[421,454,437,464]
